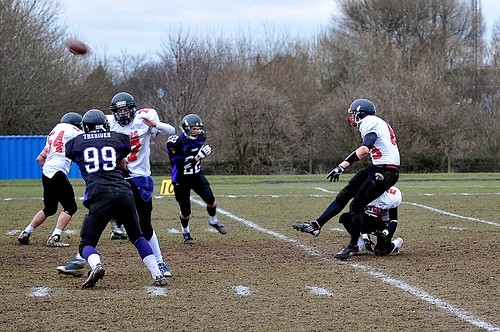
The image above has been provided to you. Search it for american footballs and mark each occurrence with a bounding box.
[65,39,87,56]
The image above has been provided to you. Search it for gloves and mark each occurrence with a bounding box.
[326,165,345,183]
[197,144,212,160]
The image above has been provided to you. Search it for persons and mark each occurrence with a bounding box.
[66,110,168,288]
[56,93,175,276]
[167,114,227,244]
[293,99,400,258]
[111,220,128,240]
[339,186,403,255]
[18,112,85,247]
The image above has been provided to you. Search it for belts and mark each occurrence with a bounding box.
[378,164,398,169]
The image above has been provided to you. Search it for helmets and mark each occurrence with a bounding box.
[83,109,110,133]
[182,113,202,140]
[61,112,82,129]
[110,92,136,124]
[347,99,376,126]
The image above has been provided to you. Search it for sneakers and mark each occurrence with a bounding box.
[182,234,194,244]
[46,236,70,247]
[157,262,173,277]
[390,236,405,255]
[56,260,85,276]
[334,244,359,260]
[81,264,105,290]
[110,230,128,240]
[208,219,226,235]
[18,231,30,245]
[363,232,375,252]
[151,278,167,286]
[292,221,321,237]
